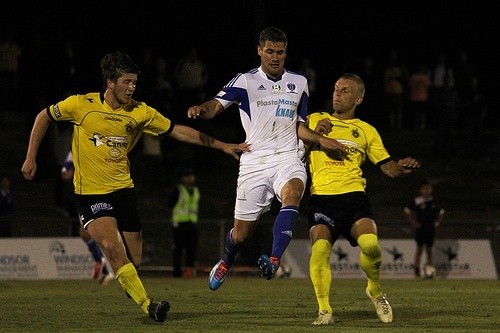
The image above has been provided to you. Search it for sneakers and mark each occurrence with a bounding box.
[365,287,393,322]
[257,254,279,281]
[89,257,105,281]
[98,271,113,286]
[208,257,233,291]
[147,296,170,322]
[311,310,335,326]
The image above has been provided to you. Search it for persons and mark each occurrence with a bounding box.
[60,155,114,286]
[0,14,499,238]
[166,162,206,278]
[188,26,353,291]
[284,72,422,326]
[404,179,446,279]
[20,48,255,322]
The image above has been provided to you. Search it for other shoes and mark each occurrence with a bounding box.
[414,266,422,279]
